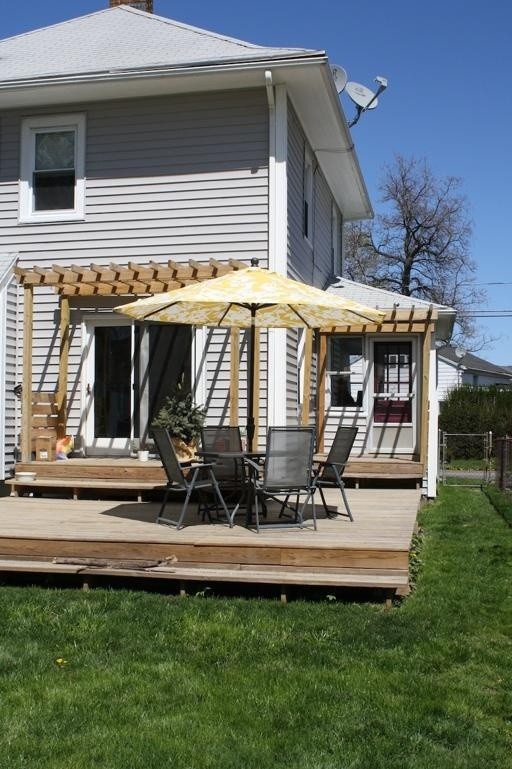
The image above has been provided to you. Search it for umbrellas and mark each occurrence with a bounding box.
[111,256,387,476]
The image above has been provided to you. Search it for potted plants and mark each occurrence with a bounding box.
[149,371,209,462]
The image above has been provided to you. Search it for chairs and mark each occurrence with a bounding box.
[149,425,360,533]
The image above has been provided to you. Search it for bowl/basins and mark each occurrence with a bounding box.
[14,472,36,482]
[137,451,149,462]
